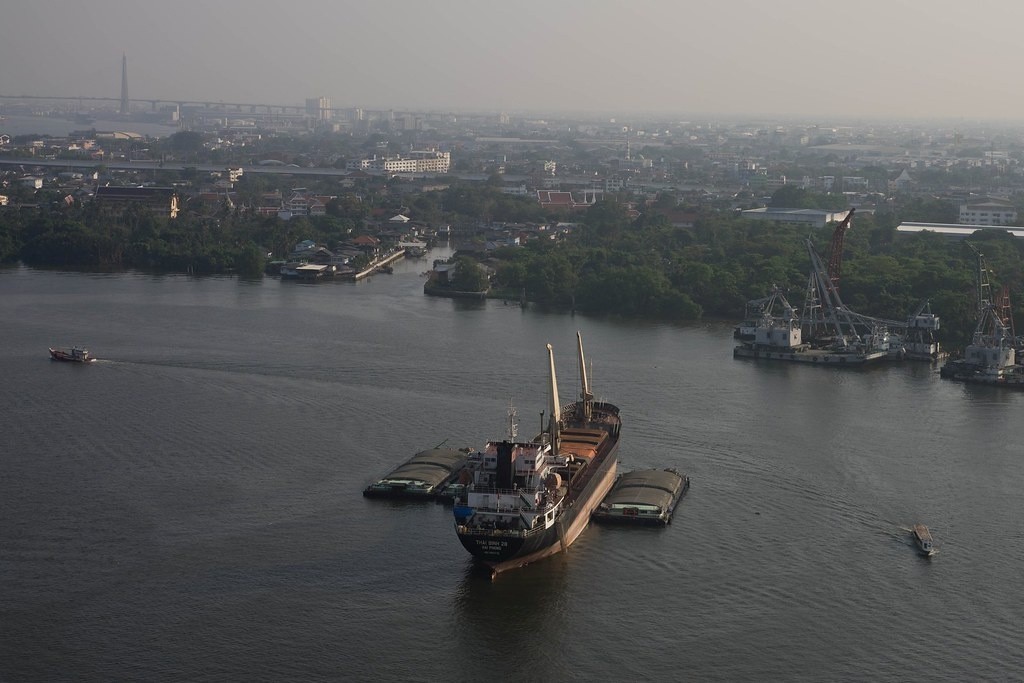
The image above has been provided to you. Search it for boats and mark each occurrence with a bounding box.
[50,346,96,364]
[396,242,427,258]
[449,330,623,573]
[912,524,934,556]
